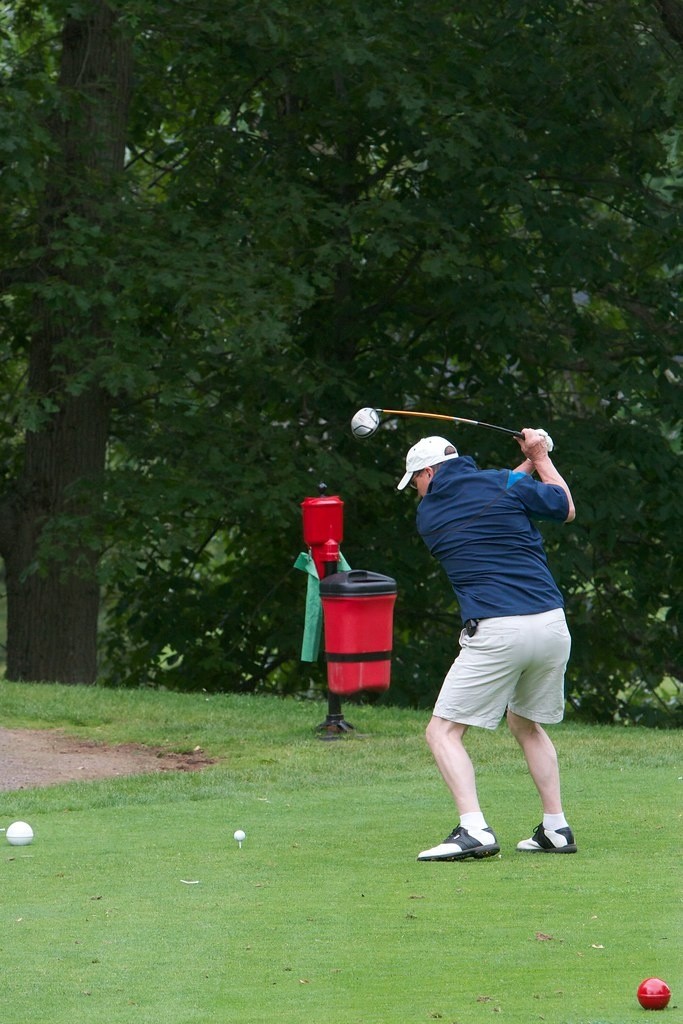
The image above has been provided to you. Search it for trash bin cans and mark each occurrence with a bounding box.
[315,570,398,695]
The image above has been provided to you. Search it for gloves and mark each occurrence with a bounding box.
[526,429,554,463]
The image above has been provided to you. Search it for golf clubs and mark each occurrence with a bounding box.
[350,406,525,441]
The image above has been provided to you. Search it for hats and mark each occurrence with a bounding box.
[397,435,460,491]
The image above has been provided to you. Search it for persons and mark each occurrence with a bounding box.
[397,428,577,860]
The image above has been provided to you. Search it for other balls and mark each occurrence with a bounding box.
[6,821,34,846]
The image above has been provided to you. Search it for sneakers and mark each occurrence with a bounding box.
[516,821,577,853]
[416,823,500,861]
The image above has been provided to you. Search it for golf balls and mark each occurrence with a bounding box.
[233,830,246,842]
